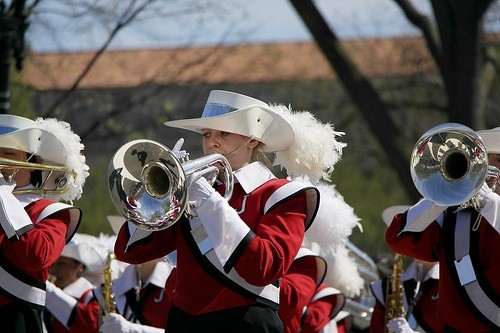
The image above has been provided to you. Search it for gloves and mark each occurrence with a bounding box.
[99,312,130,333]
[386,317,420,333]
[186,172,216,207]
[0,171,17,194]
[172,138,191,159]
[472,181,494,210]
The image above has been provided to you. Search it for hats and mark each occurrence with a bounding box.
[162,89,349,185]
[60,233,107,278]
[476,127,500,154]
[0,113,91,203]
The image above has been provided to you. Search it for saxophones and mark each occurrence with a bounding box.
[387,252,405,319]
[102,249,117,315]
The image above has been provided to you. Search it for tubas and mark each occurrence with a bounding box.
[107,139,235,232]
[411,123,500,209]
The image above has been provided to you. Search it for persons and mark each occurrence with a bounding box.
[0,90,500,333]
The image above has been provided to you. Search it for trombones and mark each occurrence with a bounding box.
[0,157,78,195]
